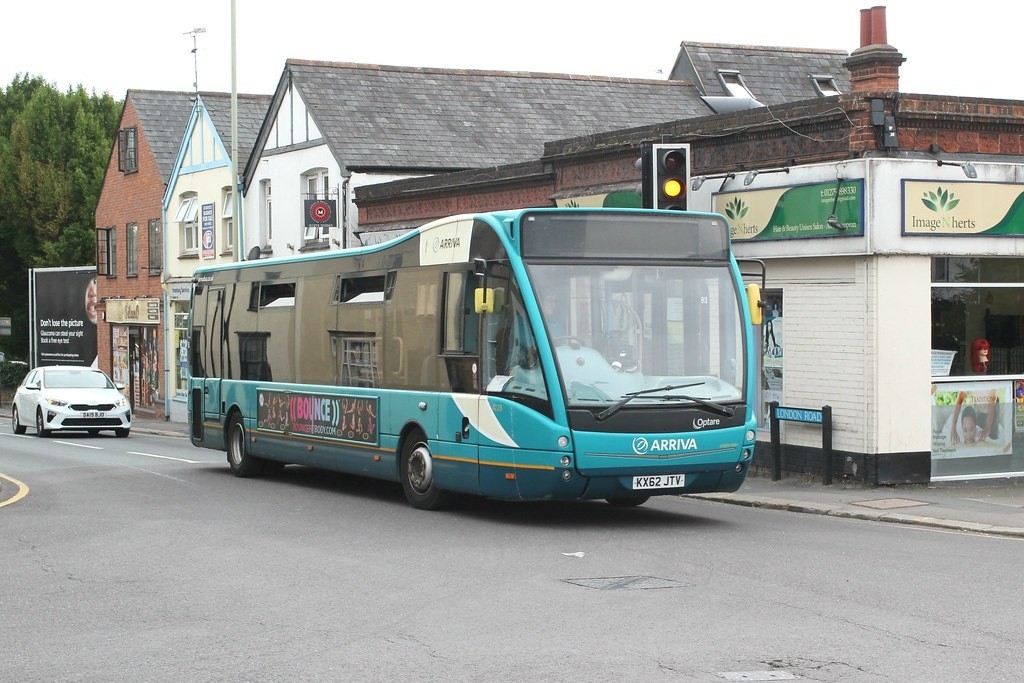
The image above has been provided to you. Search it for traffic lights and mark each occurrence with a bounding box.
[652,143,691,212]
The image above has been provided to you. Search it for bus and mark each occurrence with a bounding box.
[184,207,766,506]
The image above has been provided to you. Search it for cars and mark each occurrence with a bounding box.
[11,365,132,438]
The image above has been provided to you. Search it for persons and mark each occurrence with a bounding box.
[970,337,992,373]
[949,390,996,454]
[84,279,100,324]
[503,282,569,375]
